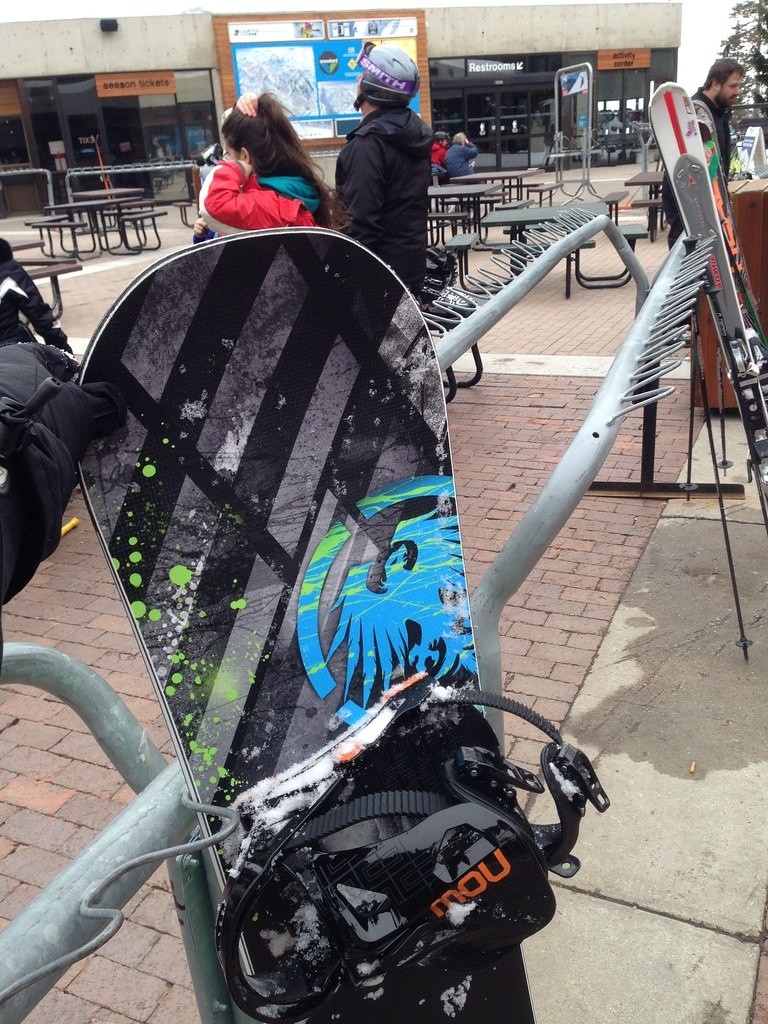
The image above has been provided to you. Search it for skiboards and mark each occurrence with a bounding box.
[646,78,768,534]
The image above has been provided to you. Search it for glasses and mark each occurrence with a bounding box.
[355,42,377,65]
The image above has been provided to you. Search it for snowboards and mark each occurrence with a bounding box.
[65,224,619,1024]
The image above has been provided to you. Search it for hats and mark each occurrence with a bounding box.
[453,133,466,143]
[435,131,450,140]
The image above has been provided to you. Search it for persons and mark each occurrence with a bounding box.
[333,41,435,298]
[193,92,353,244]
[430,131,478,186]
[662,58,746,349]
[148,135,160,159]
[0,238,127,607]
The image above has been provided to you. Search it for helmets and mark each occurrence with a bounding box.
[360,47,420,107]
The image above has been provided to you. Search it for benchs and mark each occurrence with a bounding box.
[11,182,662,320]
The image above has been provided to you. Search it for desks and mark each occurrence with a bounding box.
[625,172,666,232]
[7,240,45,259]
[424,184,504,246]
[71,188,144,235]
[44,196,142,261]
[450,170,546,202]
